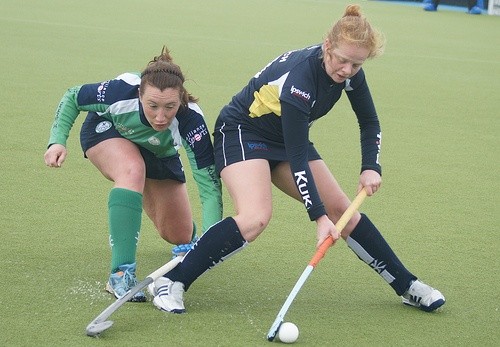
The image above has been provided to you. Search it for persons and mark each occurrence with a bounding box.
[43,44,224,303]
[145,3,447,314]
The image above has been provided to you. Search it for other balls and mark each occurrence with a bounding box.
[278,322,299,343]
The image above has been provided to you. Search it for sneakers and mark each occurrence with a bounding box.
[401,278,446,313]
[106,262,148,302]
[148,277,186,314]
[173,236,200,257]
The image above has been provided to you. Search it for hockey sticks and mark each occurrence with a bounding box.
[267,188,369,342]
[86,256,183,333]
